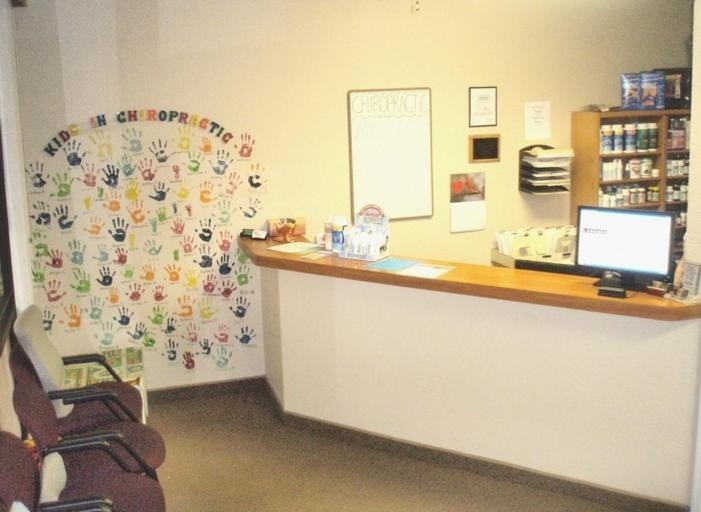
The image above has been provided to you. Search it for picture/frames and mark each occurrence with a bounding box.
[652,67,691,109]
[468,132,501,163]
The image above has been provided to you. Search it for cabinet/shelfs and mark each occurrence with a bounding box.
[571,106,691,261]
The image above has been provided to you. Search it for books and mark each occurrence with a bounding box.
[519,145,574,194]
[496,226,577,264]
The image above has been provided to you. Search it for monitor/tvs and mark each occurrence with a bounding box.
[575,206,676,292]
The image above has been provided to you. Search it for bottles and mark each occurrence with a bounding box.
[598,118,690,226]
[325,216,349,253]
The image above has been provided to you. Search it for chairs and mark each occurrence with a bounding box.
[0,302,168,511]
